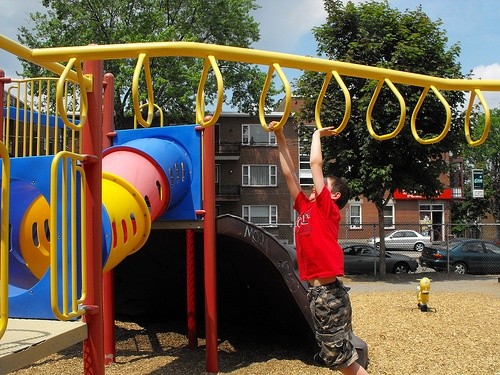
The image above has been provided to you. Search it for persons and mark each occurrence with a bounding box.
[269,121,369,375]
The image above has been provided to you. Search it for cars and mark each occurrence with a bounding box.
[367,229,432,252]
[342,243,419,276]
[419,237,500,275]
